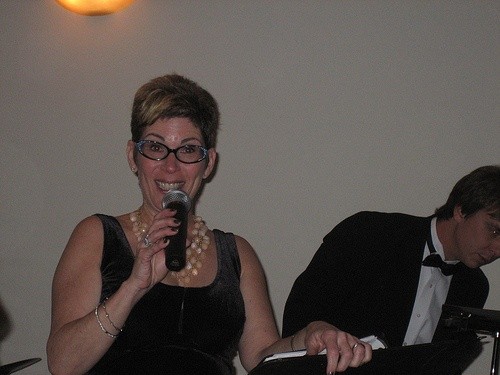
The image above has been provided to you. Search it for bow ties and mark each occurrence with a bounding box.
[422,236,460,277]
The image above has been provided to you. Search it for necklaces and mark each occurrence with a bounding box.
[130,209,211,286]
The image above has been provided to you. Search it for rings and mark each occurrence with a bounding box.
[352,342,366,351]
[144,235,150,246]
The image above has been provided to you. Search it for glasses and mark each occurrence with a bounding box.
[136,140,208,164]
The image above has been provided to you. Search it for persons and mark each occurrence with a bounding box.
[282,165,500,347]
[47,74,373,375]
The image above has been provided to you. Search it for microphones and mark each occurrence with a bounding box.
[160,190,192,271]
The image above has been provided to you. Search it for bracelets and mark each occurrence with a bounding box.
[290,333,298,351]
[94,296,123,339]
